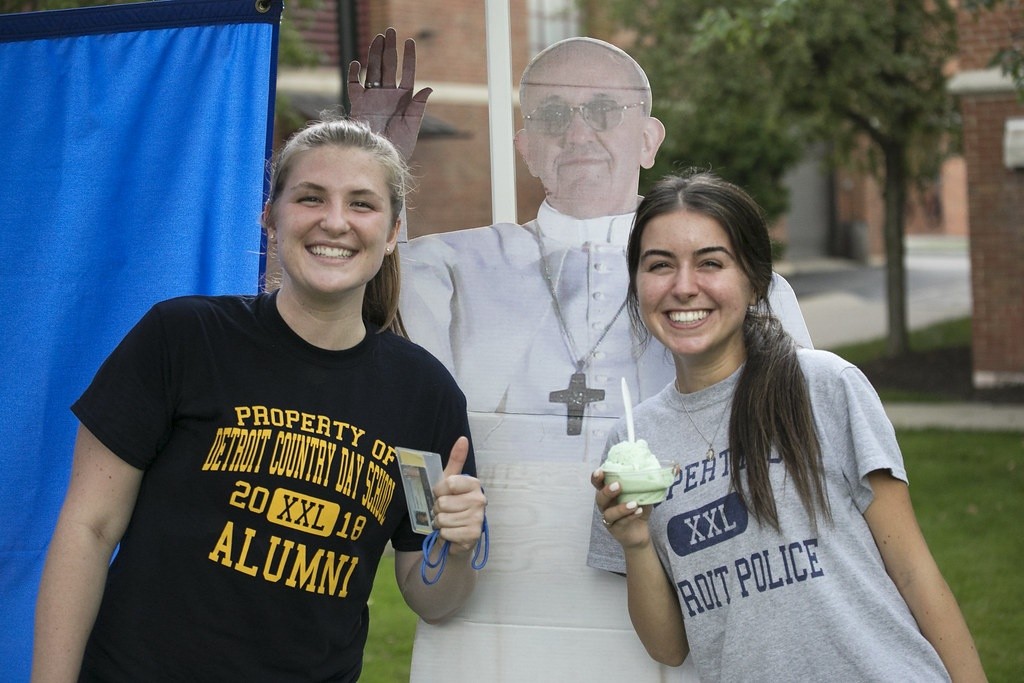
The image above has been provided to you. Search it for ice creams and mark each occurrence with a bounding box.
[601,438,674,506]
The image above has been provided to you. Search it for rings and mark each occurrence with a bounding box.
[601,514,610,527]
[364,82,379,88]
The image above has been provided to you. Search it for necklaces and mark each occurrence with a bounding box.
[675,376,736,462]
[528,218,635,438]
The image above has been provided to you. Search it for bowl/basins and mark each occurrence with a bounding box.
[604,459,676,506]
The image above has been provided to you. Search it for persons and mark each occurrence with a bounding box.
[590,177,991,682]
[347,28,815,683]
[29,124,488,682]
